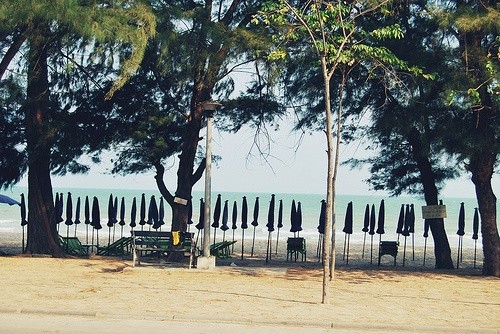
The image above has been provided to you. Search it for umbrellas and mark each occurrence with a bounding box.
[251,197,260,255]
[424,219,429,264]
[129,198,138,229]
[200,198,206,245]
[395,204,415,266]
[147,195,165,231]
[362,204,375,263]
[342,201,352,264]
[472,208,479,260]
[456,202,465,261]
[211,194,237,253]
[377,199,385,241]
[55,192,125,245]
[290,199,302,259]
[187,202,193,231]
[20,193,28,253]
[139,193,146,230]
[266,194,283,260]
[317,200,326,262]
[241,196,248,253]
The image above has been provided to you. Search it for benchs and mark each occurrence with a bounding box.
[129,231,195,268]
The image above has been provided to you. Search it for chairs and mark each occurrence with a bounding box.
[58,232,160,257]
[379,241,399,264]
[286,237,308,262]
[195,241,238,259]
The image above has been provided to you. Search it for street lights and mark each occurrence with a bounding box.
[201,102,222,258]
